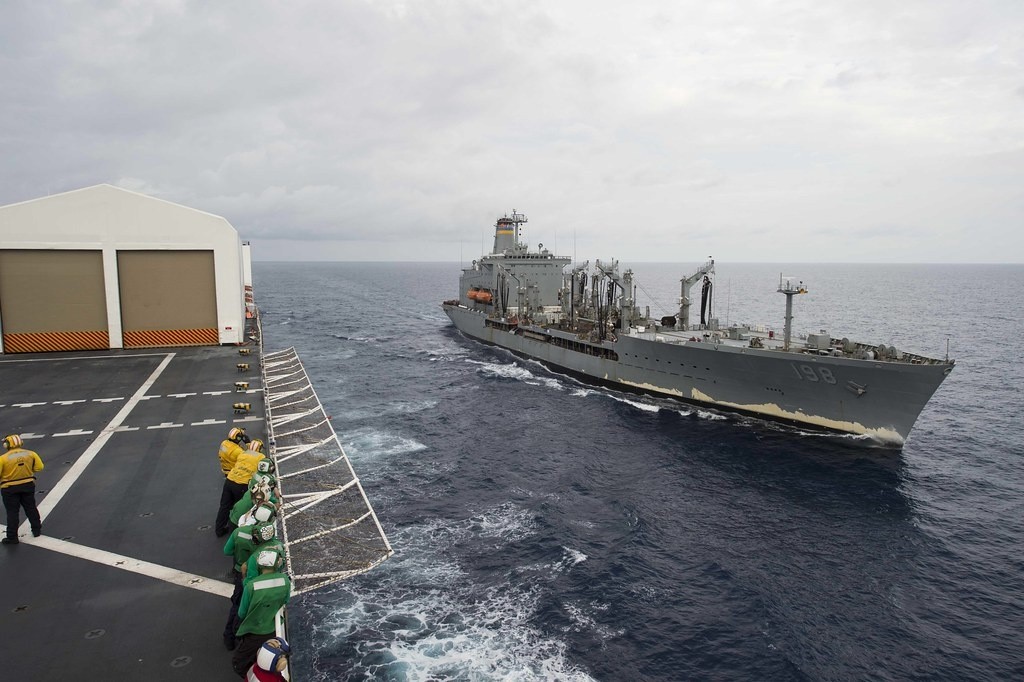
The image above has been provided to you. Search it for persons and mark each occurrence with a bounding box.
[215,428,292,682]
[0,432,45,545]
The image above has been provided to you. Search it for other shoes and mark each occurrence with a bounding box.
[227,644,237,652]
[32,529,41,537]
[2,538,19,544]
[216,527,228,537]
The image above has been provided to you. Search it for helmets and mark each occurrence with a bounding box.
[257,637,291,672]
[258,458,275,474]
[257,548,284,570]
[228,427,245,443]
[250,439,264,452]
[251,482,271,501]
[253,521,275,542]
[3,434,23,449]
[254,502,276,523]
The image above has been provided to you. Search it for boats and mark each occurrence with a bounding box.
[475,292,491,303]
[466,290,478,299]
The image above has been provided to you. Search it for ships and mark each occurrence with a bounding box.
[437,207,958,450]
